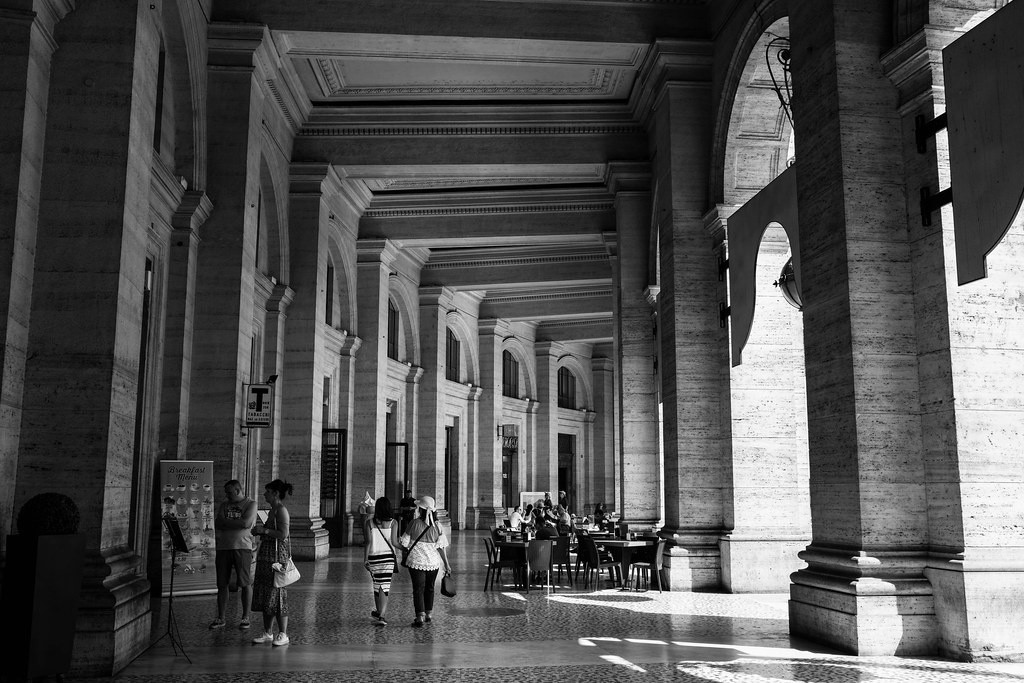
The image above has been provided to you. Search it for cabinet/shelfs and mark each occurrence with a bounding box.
[593,540,653,587]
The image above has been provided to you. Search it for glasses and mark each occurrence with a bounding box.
[518,509,521,510]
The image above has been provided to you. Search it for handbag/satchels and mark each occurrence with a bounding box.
[393,555,399,574]
[401,551,411,567]
[441,571,456,597]
[272,556,300,588]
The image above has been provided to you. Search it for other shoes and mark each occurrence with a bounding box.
[378,617,387,625]
[414,618,424,624]
[424,615,431,622]
[239,614,250,628]
[371,610,380,620]
[210,618,226,628]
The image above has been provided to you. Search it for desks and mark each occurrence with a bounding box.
[505,530,521,542]
[574,522,588,525]
[590,533,614,539]
[494,541,530,589]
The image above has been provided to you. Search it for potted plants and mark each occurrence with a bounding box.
[0,491,86,683]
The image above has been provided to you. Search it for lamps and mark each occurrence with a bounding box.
[267,374,277,385]
[771,255,803,313]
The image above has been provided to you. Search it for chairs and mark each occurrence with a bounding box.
[484,514,666,594]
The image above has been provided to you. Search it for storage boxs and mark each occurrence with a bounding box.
[523,532,531,542]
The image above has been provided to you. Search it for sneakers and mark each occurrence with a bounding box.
[254,631,274,642]
[272,632,290,645]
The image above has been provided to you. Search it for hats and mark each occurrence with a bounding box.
[559,491,566,495]
[537,502,545,508]
[414,496,437,527]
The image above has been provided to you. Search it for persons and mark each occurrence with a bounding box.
[400,496,452,628]
[364,497,406,625]
[251,480,293,646]
[400,490,417,536]
[0,493,81,683]
[209,480,258,629]
[543,492,552,528]
[510,503,571,534]
[559,490,568,514]
[534,517,560,540]
[595,502,608,525]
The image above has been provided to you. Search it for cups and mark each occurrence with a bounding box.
[626,533,637,541]
[163,483,211,505]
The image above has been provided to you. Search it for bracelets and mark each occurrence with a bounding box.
[265,529,270,535]
